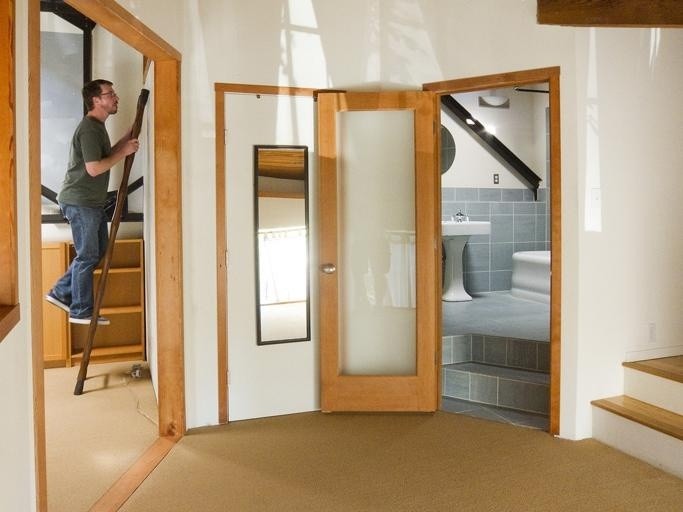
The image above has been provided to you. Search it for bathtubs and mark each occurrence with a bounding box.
[511,251,550,304]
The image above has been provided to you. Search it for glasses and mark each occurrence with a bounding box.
[97,92,116,98]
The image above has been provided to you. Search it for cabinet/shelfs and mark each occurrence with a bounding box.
[67,238,145,366]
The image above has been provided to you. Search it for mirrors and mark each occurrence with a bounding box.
[252,144,312,346]
[441,124,456,176]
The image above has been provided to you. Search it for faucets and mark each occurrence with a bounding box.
[455,213,465,222]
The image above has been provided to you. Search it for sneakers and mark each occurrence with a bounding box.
[46,289,72,314]
[69,315,111,326]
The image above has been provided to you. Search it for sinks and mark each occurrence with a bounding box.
[441,221,492,240]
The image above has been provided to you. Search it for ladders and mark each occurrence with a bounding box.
[73,89,148,395]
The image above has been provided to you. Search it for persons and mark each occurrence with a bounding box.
[43,80,140,327]
